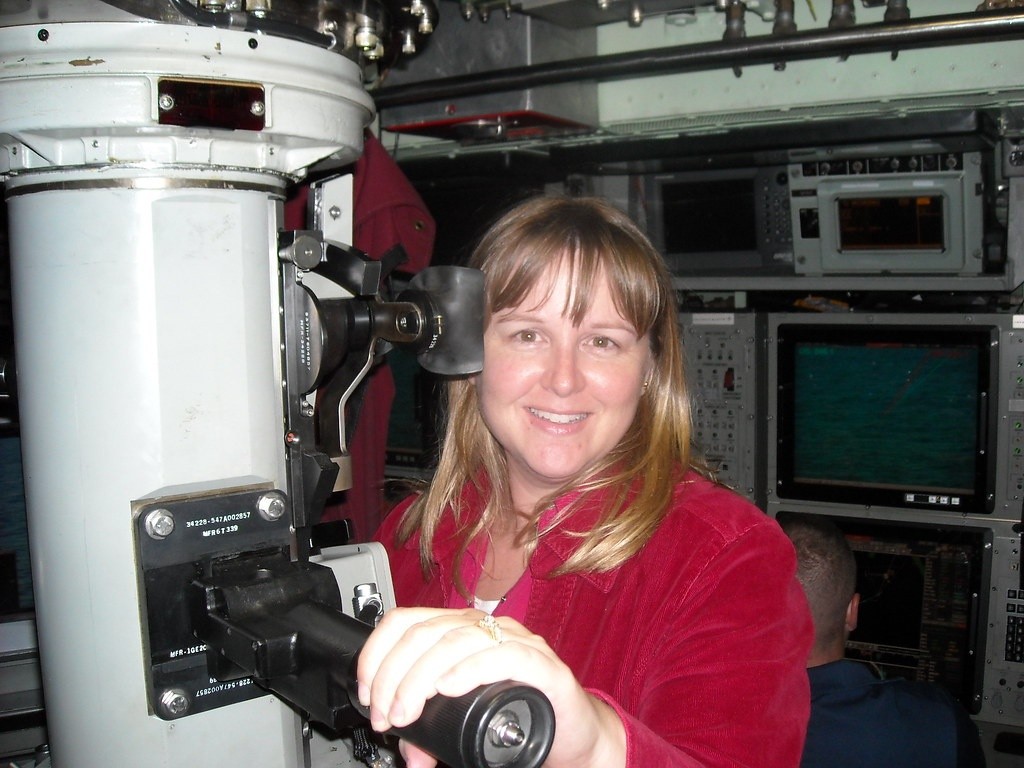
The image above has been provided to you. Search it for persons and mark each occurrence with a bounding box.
[781,512,989,768]
[274,55,438,554]
[360,191,816,768]
